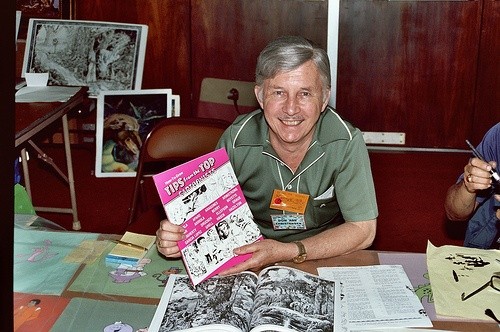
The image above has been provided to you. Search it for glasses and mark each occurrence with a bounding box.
[461,275,500,323]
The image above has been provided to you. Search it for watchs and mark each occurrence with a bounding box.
[293,241,307,264]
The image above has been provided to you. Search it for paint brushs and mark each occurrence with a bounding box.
[466,140,500,183]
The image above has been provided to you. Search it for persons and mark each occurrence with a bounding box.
[156,36,379,276]
[444,122,500,249]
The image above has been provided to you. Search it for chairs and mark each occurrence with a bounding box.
[119,118,232,234]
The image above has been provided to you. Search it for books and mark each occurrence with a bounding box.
[148,266,340,332]
[152,147,265,287]
[105,232,156,265]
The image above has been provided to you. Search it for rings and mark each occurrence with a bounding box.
[467,174,473,183]
[158,240,162,248]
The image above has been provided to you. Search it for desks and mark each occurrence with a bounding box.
[15,86,87,231]
[14,230,500,332]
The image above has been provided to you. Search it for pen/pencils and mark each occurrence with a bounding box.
[112,239,150,252]
